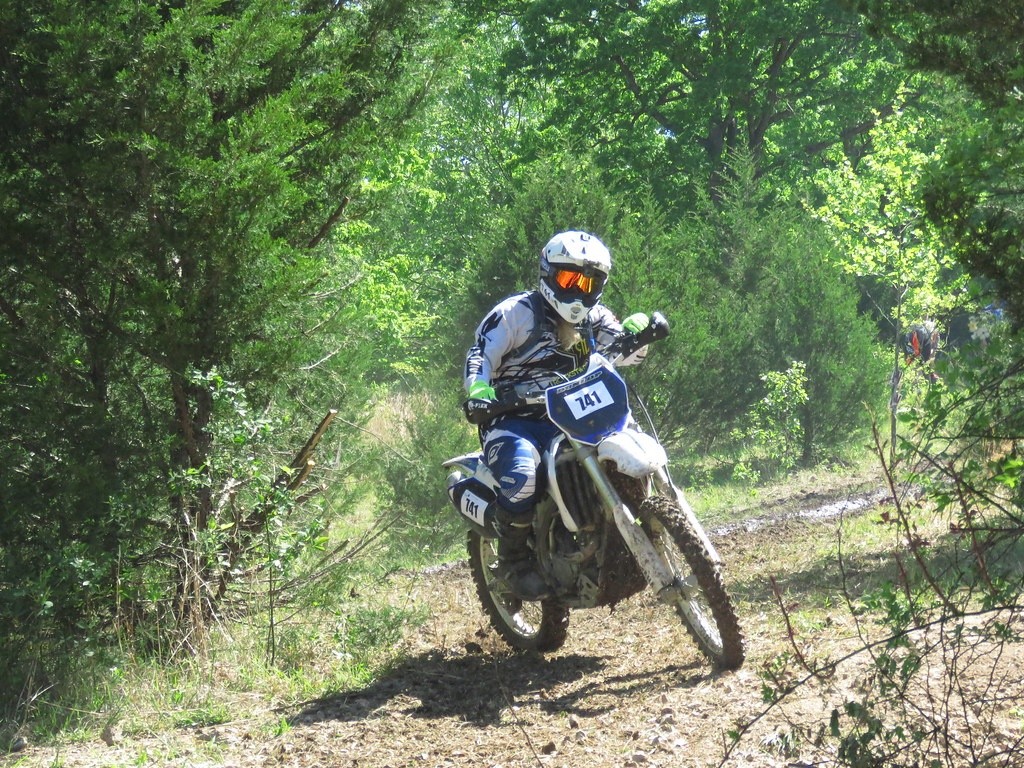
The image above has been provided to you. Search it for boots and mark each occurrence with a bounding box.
[495,501,550,600]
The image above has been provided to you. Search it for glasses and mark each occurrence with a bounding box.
[539,250,606,297]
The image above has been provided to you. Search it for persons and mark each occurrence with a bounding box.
[464,231,651,602]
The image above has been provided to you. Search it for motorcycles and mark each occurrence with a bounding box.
[443,309,749,672]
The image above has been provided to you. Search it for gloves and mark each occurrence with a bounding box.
[622,312,649,334]
[468,381,496,404]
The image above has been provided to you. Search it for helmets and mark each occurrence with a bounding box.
[538,231,612,323]
